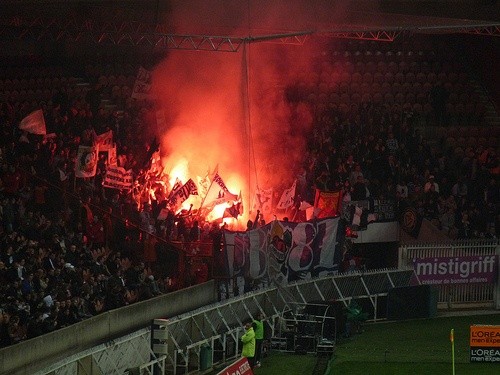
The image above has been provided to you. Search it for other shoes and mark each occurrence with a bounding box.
[254,361,263,368]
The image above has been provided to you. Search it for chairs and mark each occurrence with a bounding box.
[267,51,500,164]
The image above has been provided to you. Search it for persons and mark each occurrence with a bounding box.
[241,321,256,370]
[253,312,263,369]
[0,84,499,349]
